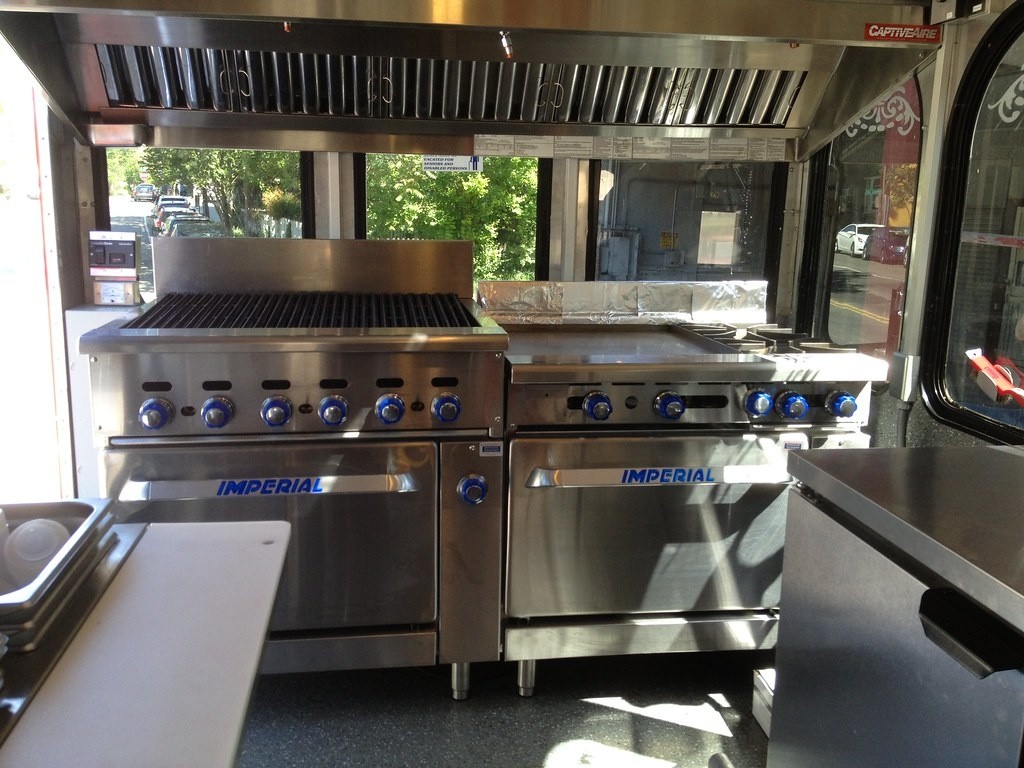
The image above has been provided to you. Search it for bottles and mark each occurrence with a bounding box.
[4,517,70,587]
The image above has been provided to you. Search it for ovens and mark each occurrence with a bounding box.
[500,368,889,698]
[79,346,503,697]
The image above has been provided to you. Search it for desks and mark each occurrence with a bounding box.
[0,519,292,768]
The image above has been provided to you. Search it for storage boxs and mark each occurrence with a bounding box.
[734,654,778,739]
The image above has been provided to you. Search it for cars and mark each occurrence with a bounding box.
[133,185,157,202]
[153,194,231,238]
[862,226,911,268]
[834,223,885,259]
